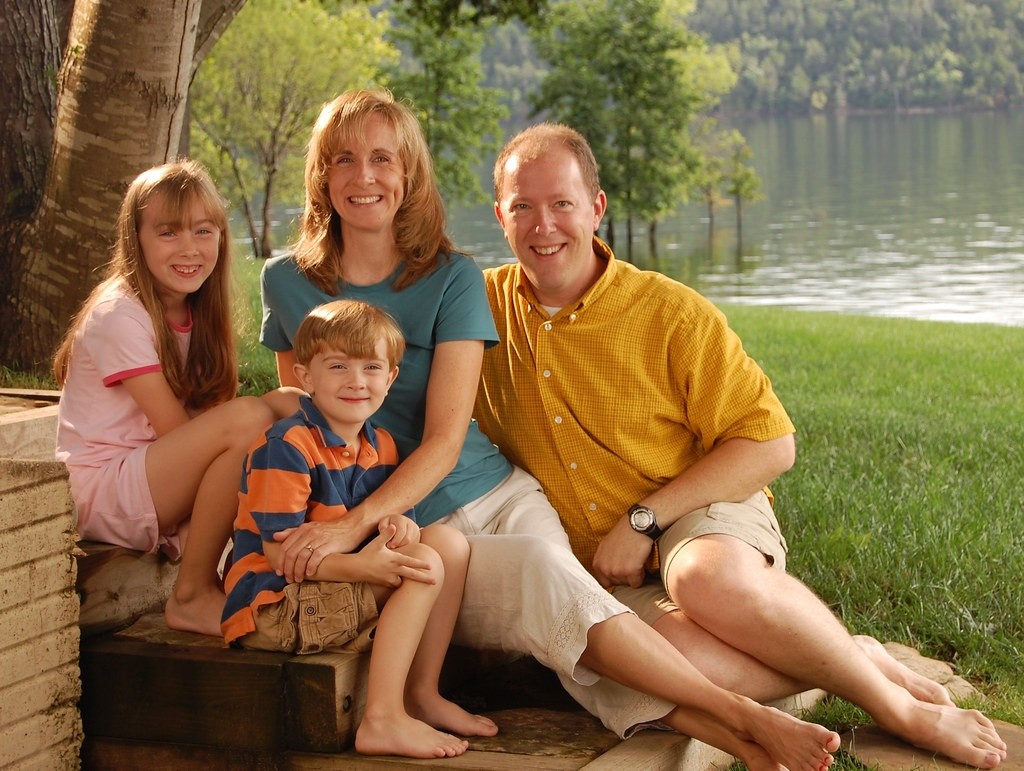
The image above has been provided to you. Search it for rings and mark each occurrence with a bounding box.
[305,543,314,553]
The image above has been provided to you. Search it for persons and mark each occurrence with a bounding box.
[220,300,500,759]
[259,90,844,771]
[54,158,314,638]
[469,124,1010,771]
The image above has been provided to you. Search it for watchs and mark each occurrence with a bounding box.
[626,505,664,539]
